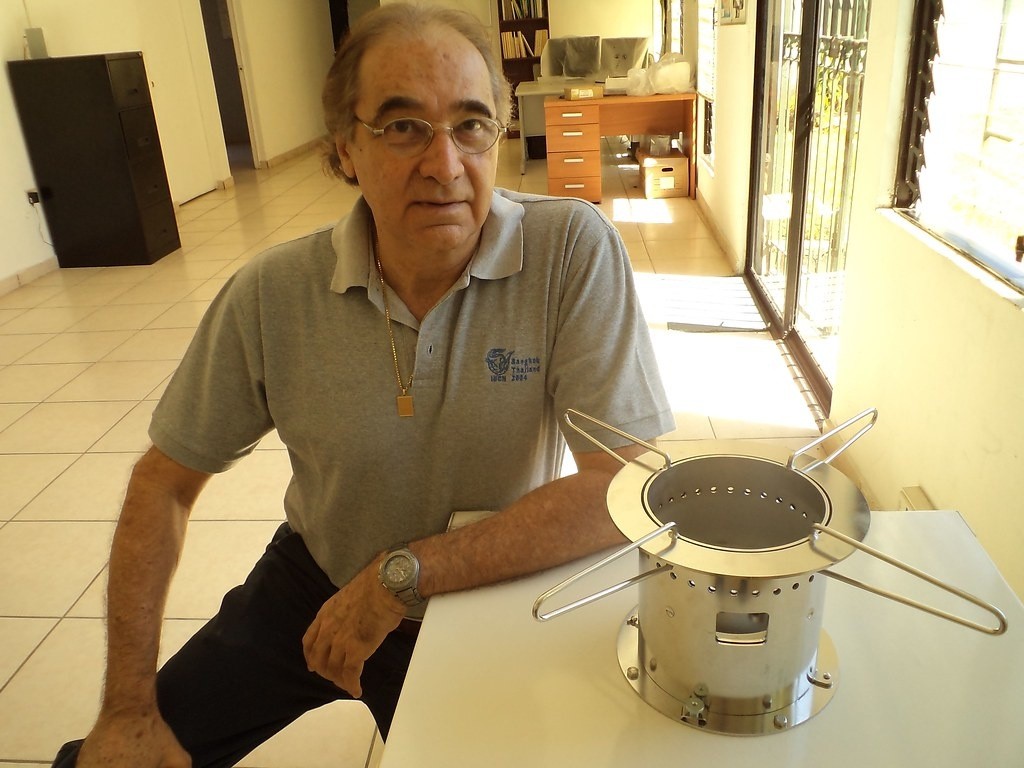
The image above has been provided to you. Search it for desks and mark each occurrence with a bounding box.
[374,509,1024,767]
[515,81,605,175]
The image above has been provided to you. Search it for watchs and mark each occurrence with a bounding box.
[377,542,425,607]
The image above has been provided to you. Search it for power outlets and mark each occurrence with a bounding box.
[28,193,40,204]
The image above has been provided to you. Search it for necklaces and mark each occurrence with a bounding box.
[374,234,414,417]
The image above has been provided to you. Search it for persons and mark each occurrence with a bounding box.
[54,0,678,768]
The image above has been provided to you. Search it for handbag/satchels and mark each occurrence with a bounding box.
[626,51,694,97]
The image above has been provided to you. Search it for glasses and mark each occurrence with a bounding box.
[351,112,507,154]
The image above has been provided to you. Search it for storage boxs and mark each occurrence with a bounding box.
[635,134,689,200]
[564,86,603,101]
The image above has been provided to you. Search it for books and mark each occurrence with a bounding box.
[501,30,548,59]
[502,0,543,21]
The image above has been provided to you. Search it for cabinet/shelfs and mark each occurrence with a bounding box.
[544,93,697,204]
[497,0,550,139]
[7,51,181,269]
